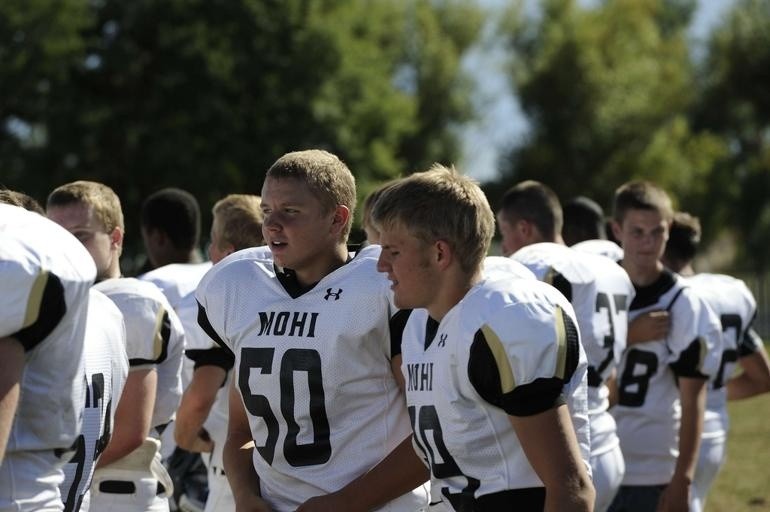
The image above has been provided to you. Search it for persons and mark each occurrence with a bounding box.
[353,194,381,260]
[0,179,266,511]
[192,145,436,512]
[369,162,601,510]
[498,174,770,510]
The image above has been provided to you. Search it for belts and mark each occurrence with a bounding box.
[100,482,163,494]
